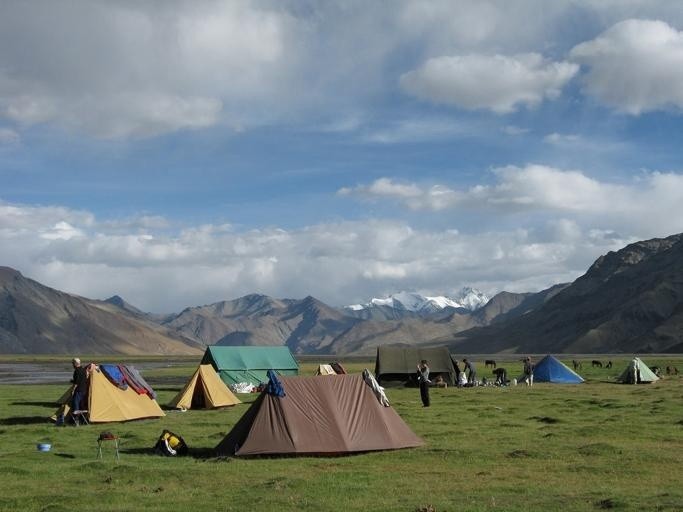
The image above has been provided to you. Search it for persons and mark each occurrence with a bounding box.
[491,367,508,387]
[416,359,431,409]
[462,358,476,382]
[523,355,535,388]
[70,355,89,423]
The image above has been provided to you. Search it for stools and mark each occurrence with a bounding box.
[73,409,90,425]
[97,438,122,460]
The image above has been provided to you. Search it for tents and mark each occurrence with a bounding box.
[200,344,299,390]
[516,353,585,384]
[165,362,242,409]
[56,361,167,423]
[375,343,462,388]
[615,356,660,384]
[210,372,425,457]
